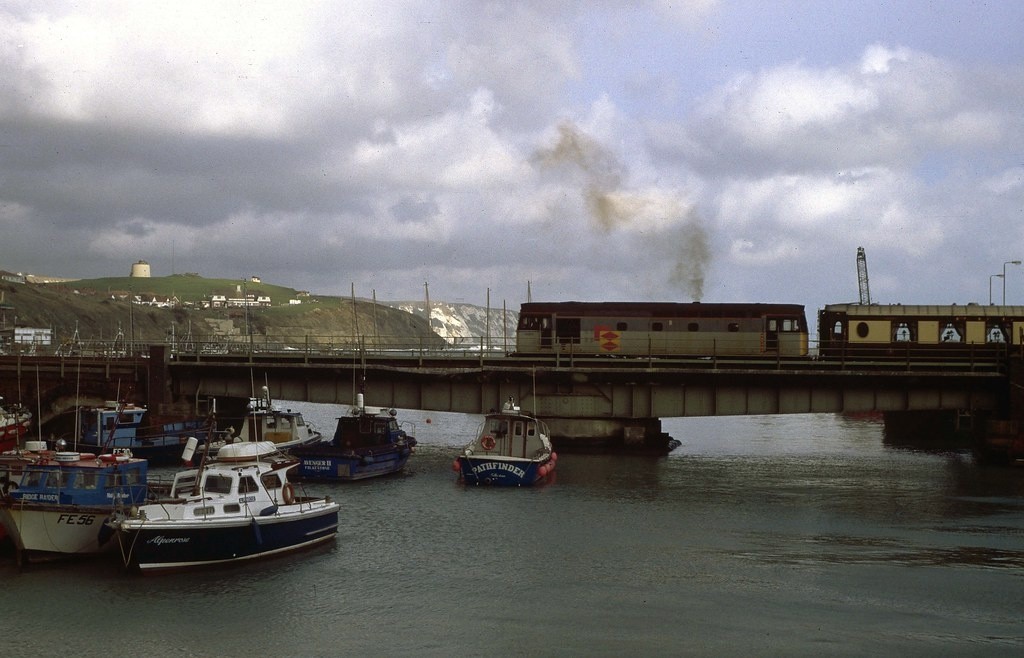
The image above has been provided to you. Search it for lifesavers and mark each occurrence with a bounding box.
[282,483,295,504]
[481,436,495,450]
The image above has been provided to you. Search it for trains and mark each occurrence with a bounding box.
[515,302,1024,362]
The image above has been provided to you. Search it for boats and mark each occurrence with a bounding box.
[55,321,231,357]
[0,357,418,565]
[454,365,559,488]
[104,279,341,571]
